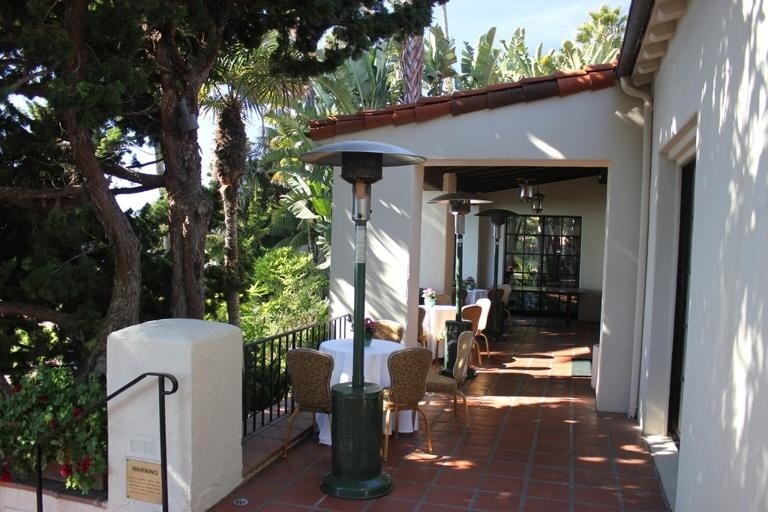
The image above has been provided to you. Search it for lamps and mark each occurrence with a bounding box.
[516,177,538,202]
[530,191,546,213]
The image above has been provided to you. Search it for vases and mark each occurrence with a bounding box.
[424,297,436,306]
[363,337,372,347]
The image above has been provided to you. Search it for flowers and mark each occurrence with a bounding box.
[422,287,436,298]
[347,313,380,340]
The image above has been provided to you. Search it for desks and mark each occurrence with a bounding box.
[511,286,586,331]
[418,304,457,360]
[314,338,420,447]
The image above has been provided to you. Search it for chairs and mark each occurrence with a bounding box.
[283,348,333,459]
[474,298,492,358]
[412,330,474,432]
[461,305,482,367]
[434,295,452,306]
[452,284,512,342]
[418,307,428,348]
[368,319,404,343]
[380,347,433,461]
